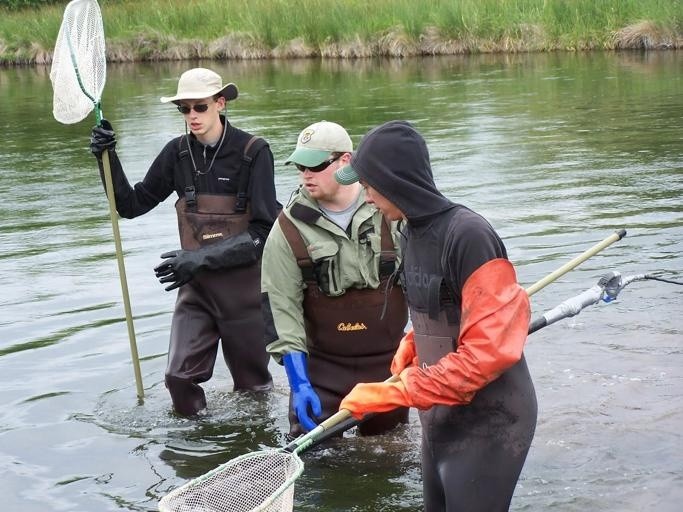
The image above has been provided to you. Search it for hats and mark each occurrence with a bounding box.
[160,68,239,105]
[285,120,353,167]
[334,164,361,185]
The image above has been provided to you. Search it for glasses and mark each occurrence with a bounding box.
[177,104,207,114]
[294,157,338,172]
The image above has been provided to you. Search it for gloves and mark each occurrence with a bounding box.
[281,351,322,431]
[339,369,414,420]
[390,329,419,375]
[89,119,134,219]
[154,233,259,292]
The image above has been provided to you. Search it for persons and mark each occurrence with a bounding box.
[337,118,538,511]
[257,118,409,444]
[89,67,283,418]
